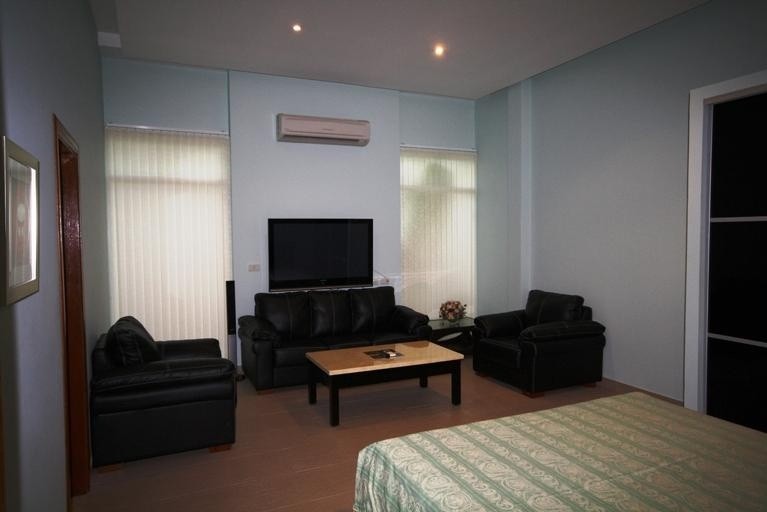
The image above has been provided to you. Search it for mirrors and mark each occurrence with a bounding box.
[1,135,40,306]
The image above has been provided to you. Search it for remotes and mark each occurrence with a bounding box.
[382,348,396,357]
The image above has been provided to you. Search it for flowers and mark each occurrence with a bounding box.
[437,300,467,324]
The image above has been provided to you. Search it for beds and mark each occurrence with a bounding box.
[351,391,766,511]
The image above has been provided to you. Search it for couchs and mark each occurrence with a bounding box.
[237,287,432,395]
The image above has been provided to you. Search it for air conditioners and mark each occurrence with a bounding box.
[275,113,370,147]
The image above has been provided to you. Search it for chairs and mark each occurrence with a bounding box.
[88,316,236,473]
[472,290,605,398]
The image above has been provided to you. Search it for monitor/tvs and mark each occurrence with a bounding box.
[268,218,373,293]
